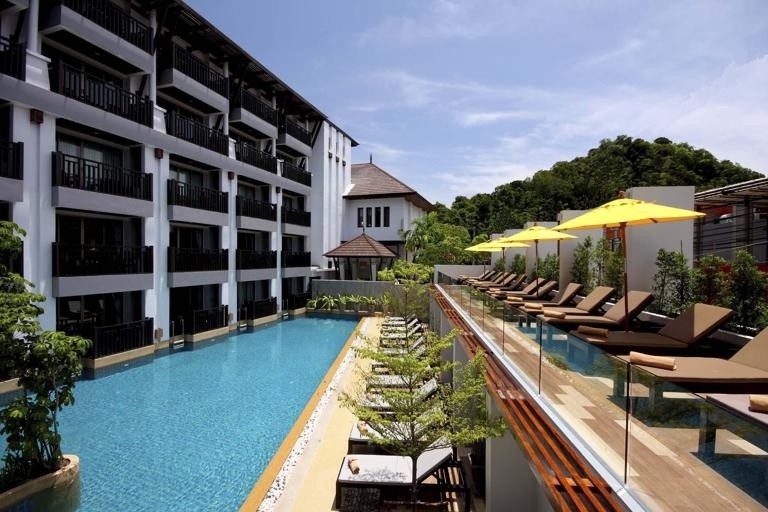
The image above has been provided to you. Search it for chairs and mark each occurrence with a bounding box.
[335,309,474,512]
[564,303,732,370]
[460,271,510,289]
[502,283,581,319]
[488,280,556,310]
[698,391,768,457]
[613,326,768,426]
[518,285,614,330]
[483,278,545,305]
[477,275,527,299]
[471,274,516,294]
[536,291,654,347]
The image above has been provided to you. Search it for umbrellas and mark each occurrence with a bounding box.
[552,191,707,332]
[464,223,580,299]
